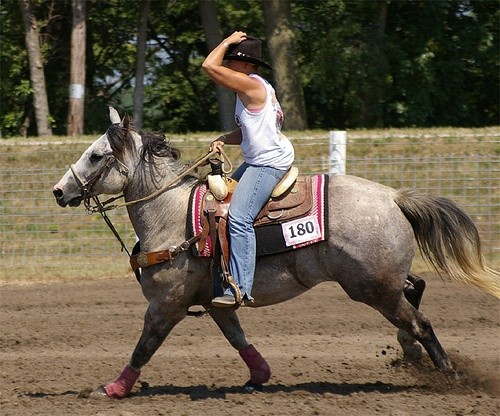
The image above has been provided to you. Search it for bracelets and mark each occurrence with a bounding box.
[220,40,229,49]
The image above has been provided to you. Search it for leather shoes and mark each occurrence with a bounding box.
[211,295,249,308]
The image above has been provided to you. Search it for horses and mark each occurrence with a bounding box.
[52,105,500,400]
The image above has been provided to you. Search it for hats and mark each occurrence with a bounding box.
[223,35,272,70]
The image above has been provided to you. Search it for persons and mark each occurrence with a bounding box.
[201,30,295,306]
